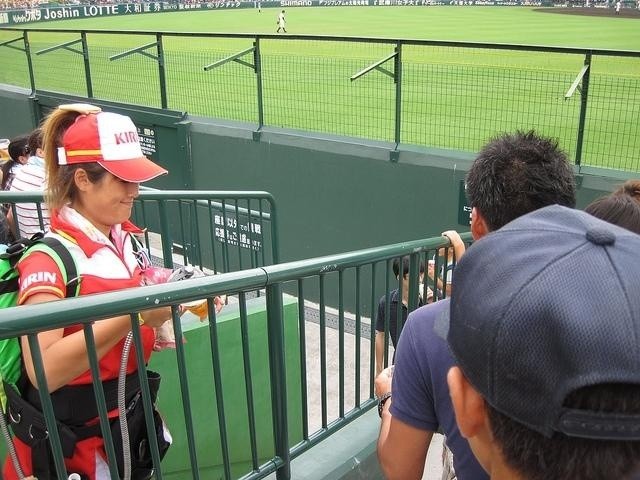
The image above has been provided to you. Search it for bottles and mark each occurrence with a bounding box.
[417,284,434,304]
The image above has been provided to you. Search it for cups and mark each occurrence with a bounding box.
[167,267,208,321]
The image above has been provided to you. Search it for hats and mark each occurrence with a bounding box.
[56,112,170,185]
[446,203,640,441]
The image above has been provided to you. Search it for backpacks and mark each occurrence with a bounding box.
[0,226,146,425]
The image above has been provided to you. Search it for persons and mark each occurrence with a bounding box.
[1,103,224,480]
[0,0,640,32]
[374,128,640,480]
